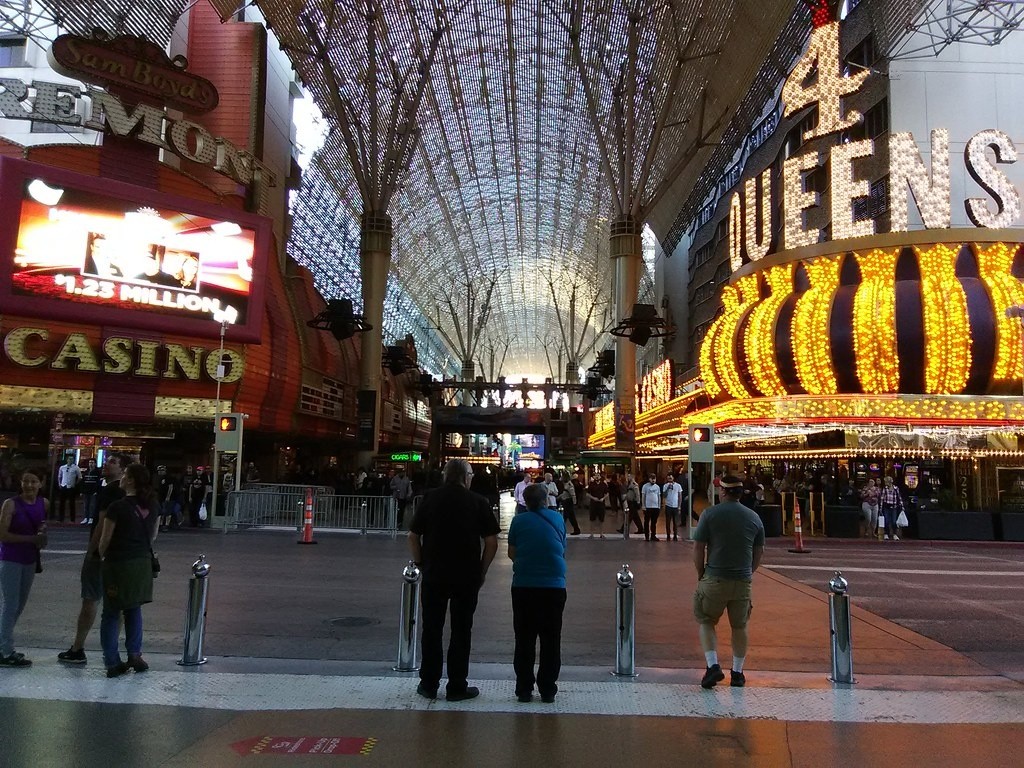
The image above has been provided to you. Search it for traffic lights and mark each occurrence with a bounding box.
[220,417,237,431]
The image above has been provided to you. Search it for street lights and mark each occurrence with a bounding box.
[212,305,238,414]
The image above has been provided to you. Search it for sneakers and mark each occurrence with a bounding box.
[702,663,725,689]
[0,651,32,667]
[58,645,87,663]
[731,670,745,686]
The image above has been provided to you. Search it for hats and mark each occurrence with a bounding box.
[197,467,203,470]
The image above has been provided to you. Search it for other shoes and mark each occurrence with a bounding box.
[519,690,531,702]
[872,532,878,538]
[128,655,148,671]
[893,535,899,541]
[80,517,87,524]
[884,534,889,540]
[541,692,555,703]
[107,662,128,677]
[417,684,438,698]
[447,686,479,701]
[87,518,93,524]
[162,525,169,532]
[864,530,868,536]
[570,513,687,542]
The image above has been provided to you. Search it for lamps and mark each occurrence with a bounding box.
[382,346,442,392]
[576,350,615,400]
[306,299,373,340]
[610,304,676,347]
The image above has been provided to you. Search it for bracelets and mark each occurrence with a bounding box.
[413,562,422,567]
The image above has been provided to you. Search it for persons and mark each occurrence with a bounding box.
[534,472,545,483]
[246,462,261,483]
[151,463,214,532]
[557,471,581,535]
[0,467,51,670]
[542,473,558,511]
[616,472,683,540]
[585,473,609,538]
[58,454,83,522]
[572,469,588,506]
[692,475,766,688]
[223,462,234,515]
[713,470,905,540]
[57,452,133,663]
[514,473,532,514]
[354,465,412,529]
[678,470,699,527]
[81,458,103,524]
[607,474,621,515]
[508,483,567,703]
[408,459,502,703]
[99,462,161,677]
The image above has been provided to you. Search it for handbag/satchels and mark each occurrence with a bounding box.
[152,554,160,578]
[896,510,908,527]
[878,513,885,527]
[35,549,43,574]
[199,502,207,520]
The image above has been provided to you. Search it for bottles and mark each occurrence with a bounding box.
[669,483,673,491]
[36,521,48,549]
[397,490,400,498]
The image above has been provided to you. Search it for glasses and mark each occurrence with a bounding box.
[468,473,475,477]
[66,467,69,472]
